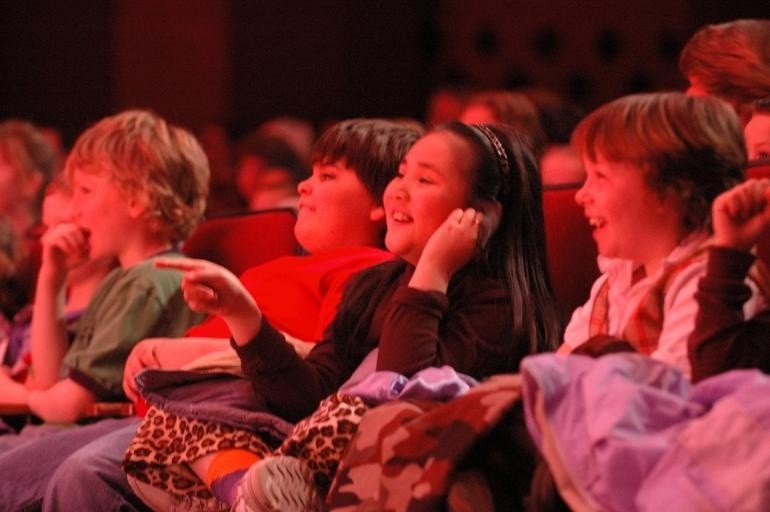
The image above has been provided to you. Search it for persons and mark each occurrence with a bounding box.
[1,19,770,512]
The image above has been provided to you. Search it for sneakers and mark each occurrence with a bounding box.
[229,457,322,511]
